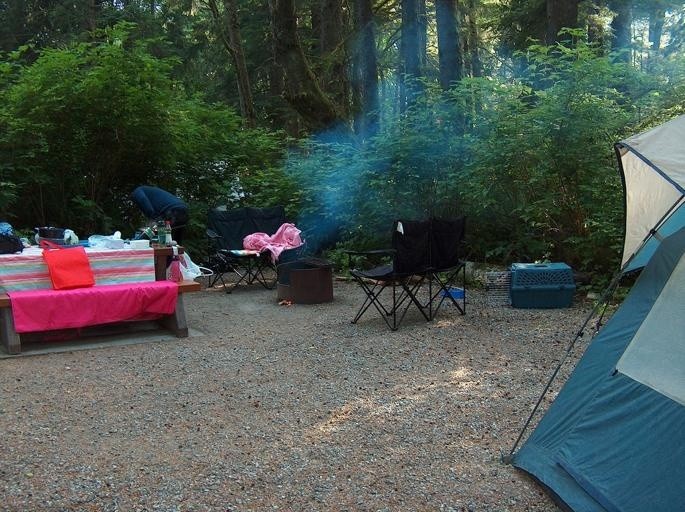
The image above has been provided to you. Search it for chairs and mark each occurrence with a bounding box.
[345,214,467,332]
[209,203,307,295]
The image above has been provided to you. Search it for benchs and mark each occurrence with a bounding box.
[0,282,203,355]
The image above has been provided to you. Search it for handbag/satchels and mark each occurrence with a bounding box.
[179,251,213,282]
[40,240,97,291]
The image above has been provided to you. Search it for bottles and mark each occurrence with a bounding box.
[157,220,174,247]
[137,225,157,240]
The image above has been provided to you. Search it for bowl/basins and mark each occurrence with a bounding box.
[130,240,150,250]
[39,227,65,248]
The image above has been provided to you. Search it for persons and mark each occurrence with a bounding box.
[129,184,189,246]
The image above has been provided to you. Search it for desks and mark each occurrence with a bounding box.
[0,237,184,292]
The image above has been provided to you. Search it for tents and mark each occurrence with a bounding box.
[499,111,685,512]
